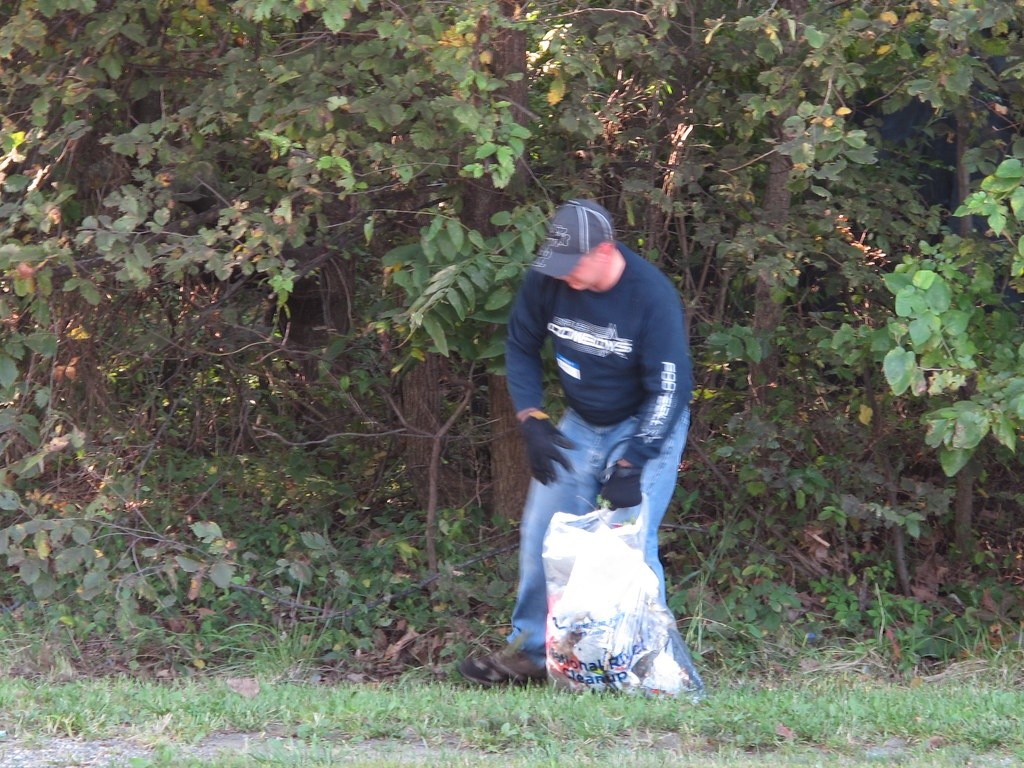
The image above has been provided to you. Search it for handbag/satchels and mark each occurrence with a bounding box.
[541,493,706,704]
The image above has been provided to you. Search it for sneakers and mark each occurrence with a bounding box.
[459,643,547,687]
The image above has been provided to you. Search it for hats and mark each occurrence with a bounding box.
[529,198,614,277]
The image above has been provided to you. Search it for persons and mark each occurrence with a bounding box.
[458,199,695,688]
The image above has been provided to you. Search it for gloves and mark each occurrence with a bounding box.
[602,458,642,511]
[521,411,575,486]
